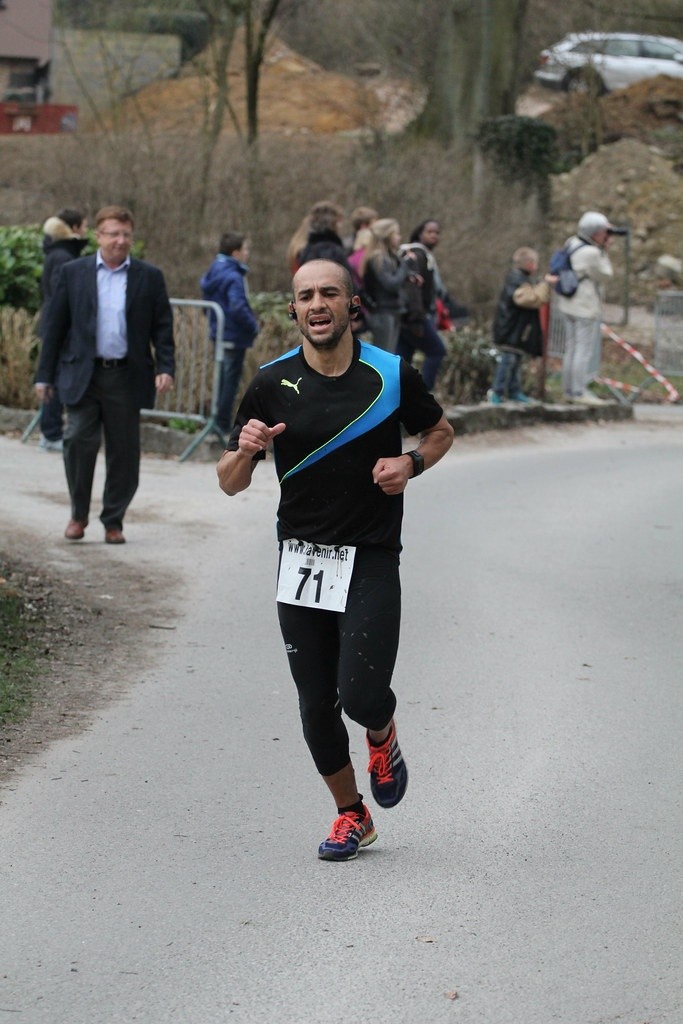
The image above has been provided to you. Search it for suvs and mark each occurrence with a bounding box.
[535,33,683,100]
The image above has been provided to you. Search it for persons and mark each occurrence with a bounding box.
[287,202,447,391]
[484,246,560,404]
[547,211,631,406]
[197,228,266,446]
[214,259,456,863]
[39,207,90,450]
[34,206,175,544]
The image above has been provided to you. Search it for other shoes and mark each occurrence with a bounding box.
[490,392,502,402]
[561,392,576,403]
[508,390,529,402]
[580,392,617,406]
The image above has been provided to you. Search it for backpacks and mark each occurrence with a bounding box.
[549,237,589,298]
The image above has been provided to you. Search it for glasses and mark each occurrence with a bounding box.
[101,231,133,238]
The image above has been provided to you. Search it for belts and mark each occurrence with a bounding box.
[94,356,124,369]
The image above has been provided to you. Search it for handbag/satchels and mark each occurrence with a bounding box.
[40,386,64,441]
[388,247,425,322]
[434,298,453,330]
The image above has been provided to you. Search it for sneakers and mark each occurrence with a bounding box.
[365,718,408,808]
[318,803,378,861]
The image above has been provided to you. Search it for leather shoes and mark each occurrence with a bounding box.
[106,528,125,542]
[65,519,88,539]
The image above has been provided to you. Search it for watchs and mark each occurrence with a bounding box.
[405,450,425,479]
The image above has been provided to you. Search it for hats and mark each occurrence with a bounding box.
[369,218,396,239]
[579,211,612,237]
[352,206,378,221]
